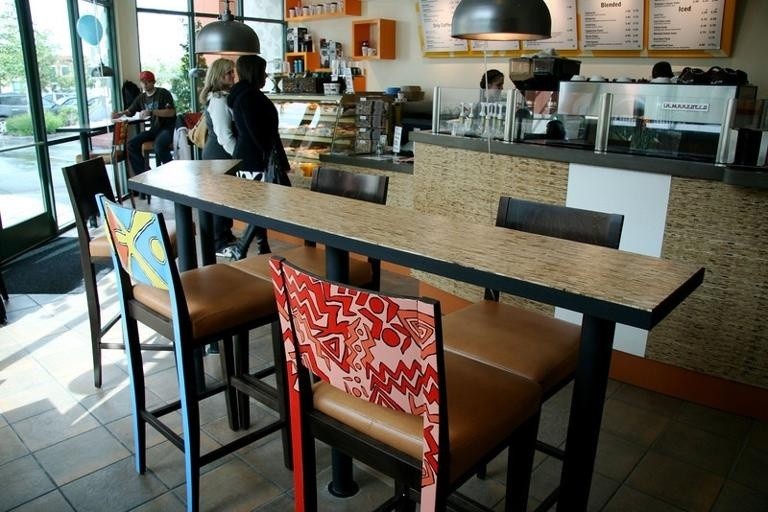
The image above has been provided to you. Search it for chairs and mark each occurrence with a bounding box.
[75,120,136,210]
[440,195,625,481]
[139,141,175,204]
[228,168,389,431]
[60,156,196,389]
[94,192,294,512]
[268,255,541,512]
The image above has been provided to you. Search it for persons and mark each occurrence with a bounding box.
[202,58,239,259]
[472,69,507,120]
[226,54,292,261]
[111,71,177,176]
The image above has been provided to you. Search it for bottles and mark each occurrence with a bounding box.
[362,40,369,56]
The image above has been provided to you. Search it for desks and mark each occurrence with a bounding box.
[127,159,706,510]
[54,118,151,161]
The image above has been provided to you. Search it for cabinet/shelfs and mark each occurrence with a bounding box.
[268,94,402,158]
[283,0,395,94]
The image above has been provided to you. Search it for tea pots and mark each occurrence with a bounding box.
[279,61,290,74]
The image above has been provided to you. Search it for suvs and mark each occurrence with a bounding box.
[0,93,78,136]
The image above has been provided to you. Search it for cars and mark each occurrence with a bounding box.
[41,87,113,122]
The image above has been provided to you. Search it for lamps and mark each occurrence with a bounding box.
[450,0,552,41]
[194,0,260,56]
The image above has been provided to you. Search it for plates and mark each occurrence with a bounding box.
[569,74,673,84]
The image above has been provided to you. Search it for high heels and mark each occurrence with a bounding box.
[229,247,241,261]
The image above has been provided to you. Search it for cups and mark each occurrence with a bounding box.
[368,48,376,56]
[323,83,341,94]
[397,93,404,99]
[289,1,342,18]
[350,68,361,75]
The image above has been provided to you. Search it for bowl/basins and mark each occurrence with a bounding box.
[385,87,401,95]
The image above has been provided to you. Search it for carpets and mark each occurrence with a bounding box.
[1,237,115,295]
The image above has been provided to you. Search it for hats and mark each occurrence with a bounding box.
[140,71,154,81]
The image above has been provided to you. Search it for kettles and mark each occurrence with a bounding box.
[294,59,304,74]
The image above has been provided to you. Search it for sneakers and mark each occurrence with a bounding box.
[216,246,233,257]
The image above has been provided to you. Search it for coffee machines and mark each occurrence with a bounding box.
[509,57,581,141]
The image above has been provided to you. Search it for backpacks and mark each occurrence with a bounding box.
[122,80,140,109]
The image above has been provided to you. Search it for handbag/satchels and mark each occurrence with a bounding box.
[188,113,207,148]
[266,157,291,187]
[677,65,747,85]
